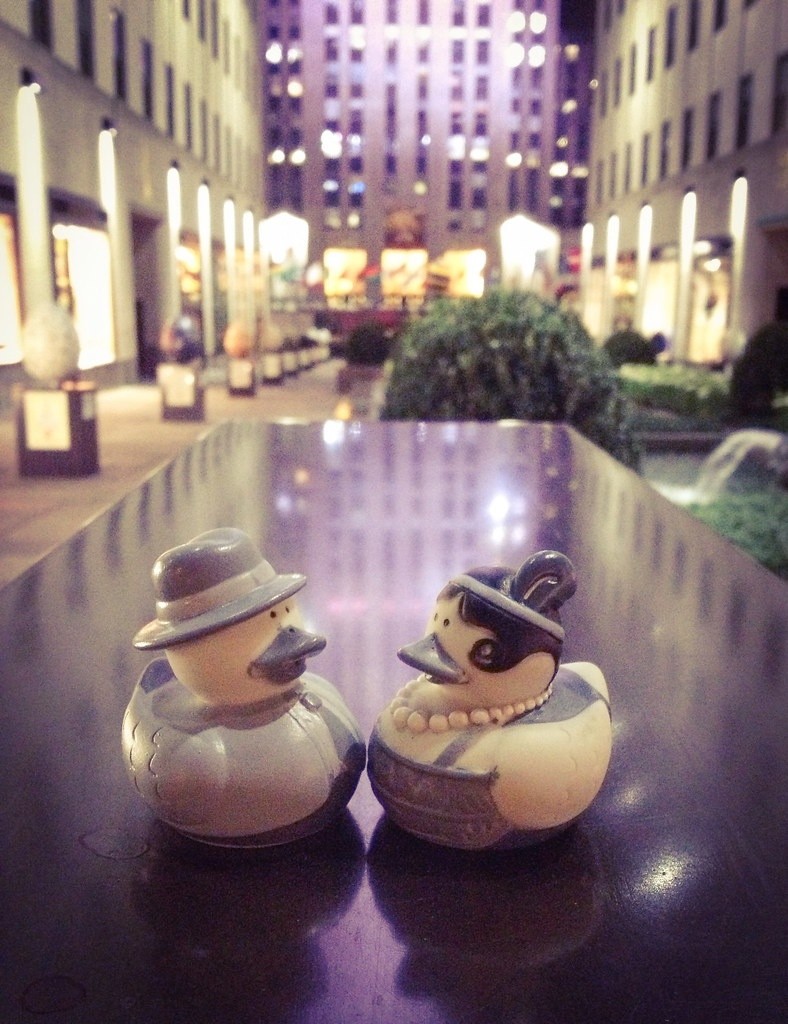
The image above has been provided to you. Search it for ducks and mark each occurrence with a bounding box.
[365,547,614,848]
[121,526,365,851]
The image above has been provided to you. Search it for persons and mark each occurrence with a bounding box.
[728,285,788,430]
[171,301,209,371]
[604,312,665,367]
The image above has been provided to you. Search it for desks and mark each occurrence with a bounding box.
[0,419,788,1024]
